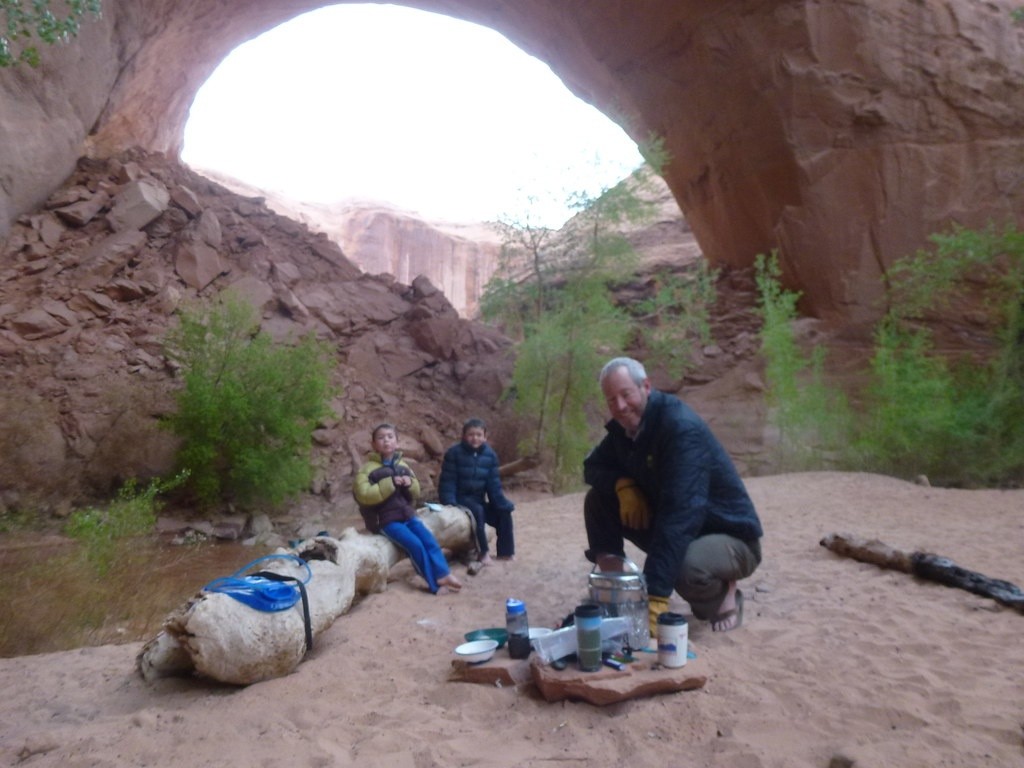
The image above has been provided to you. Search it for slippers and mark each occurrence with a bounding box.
[711,588,744,632]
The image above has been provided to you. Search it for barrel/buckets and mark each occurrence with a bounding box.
[589,555,650,651]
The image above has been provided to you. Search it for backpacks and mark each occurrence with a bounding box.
[204,555,312,613]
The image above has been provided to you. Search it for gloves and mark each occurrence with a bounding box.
[649,594,673,639]
[614,477,655,531]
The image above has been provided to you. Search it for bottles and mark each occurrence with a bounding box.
[506,598,531,658]
[574,605,603,672]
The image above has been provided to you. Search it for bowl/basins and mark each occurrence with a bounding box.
[528,628,551,647]
[455,640,499,663]
[465,628,508,648]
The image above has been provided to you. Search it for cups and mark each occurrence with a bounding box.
[657,613,689,668]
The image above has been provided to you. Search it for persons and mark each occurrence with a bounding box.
[352,423,463,596]
[583,357,765,633]
[438,418,517,566]
